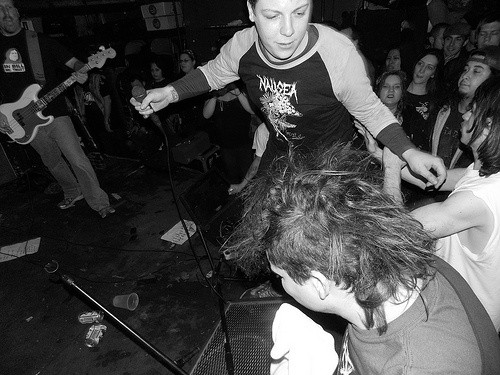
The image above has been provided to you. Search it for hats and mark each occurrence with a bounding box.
[468,48,500,70]
[443,22,470,40]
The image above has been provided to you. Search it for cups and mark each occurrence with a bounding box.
[113,293,139,311]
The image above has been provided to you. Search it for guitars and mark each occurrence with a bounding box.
[0,46,116,145]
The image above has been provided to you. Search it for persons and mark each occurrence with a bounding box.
[354,77,500,336]
[220,139,500,375]
[0,0,500,219]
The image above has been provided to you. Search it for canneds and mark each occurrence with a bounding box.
[84,323,107,347]
[78,310,104,324]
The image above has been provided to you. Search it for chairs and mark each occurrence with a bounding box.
[125,39,179,66]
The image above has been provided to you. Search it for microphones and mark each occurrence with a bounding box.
[132,85,162,127]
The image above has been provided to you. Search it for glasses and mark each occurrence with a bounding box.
[177,59,193,63]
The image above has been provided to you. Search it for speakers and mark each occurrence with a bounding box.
[179,172,243,248]
[190,297,300,375]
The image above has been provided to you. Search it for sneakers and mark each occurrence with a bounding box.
[240,280,284,299]
[98,206,116,218]
[57,191,85,209]
